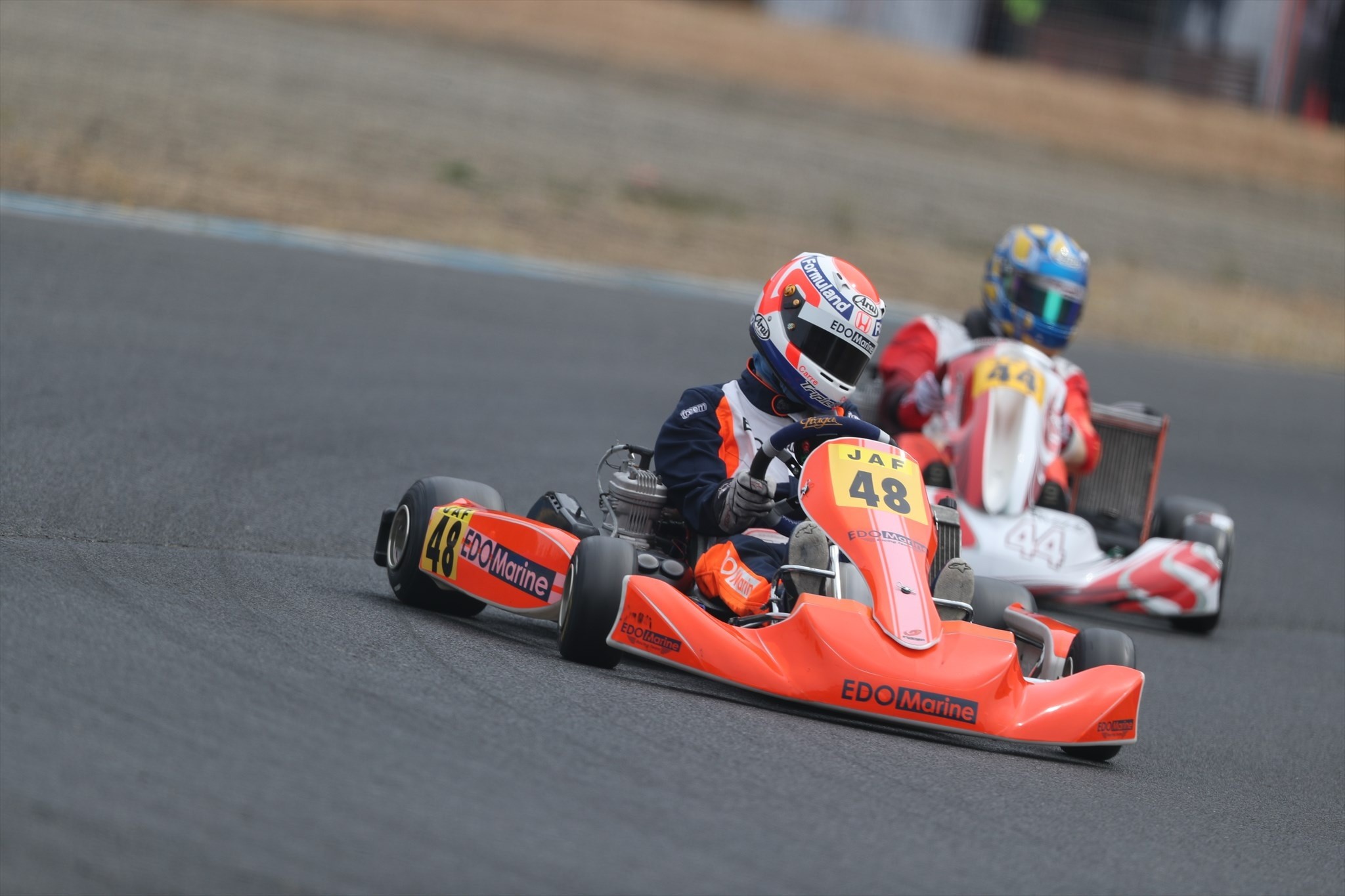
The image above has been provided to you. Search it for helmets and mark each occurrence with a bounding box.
[748,251,887,415]
[981,223,1091,356]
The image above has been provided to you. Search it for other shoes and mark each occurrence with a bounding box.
[930,557,976,621]
[923,461,952,489]
[780,521,830,603]
[1035,481,1069,512]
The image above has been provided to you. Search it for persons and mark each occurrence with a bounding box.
[653,252,975,625]
[878,224,1100,512]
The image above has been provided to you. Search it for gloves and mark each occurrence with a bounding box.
[713,470,775,535]
[914,369,944,415]
